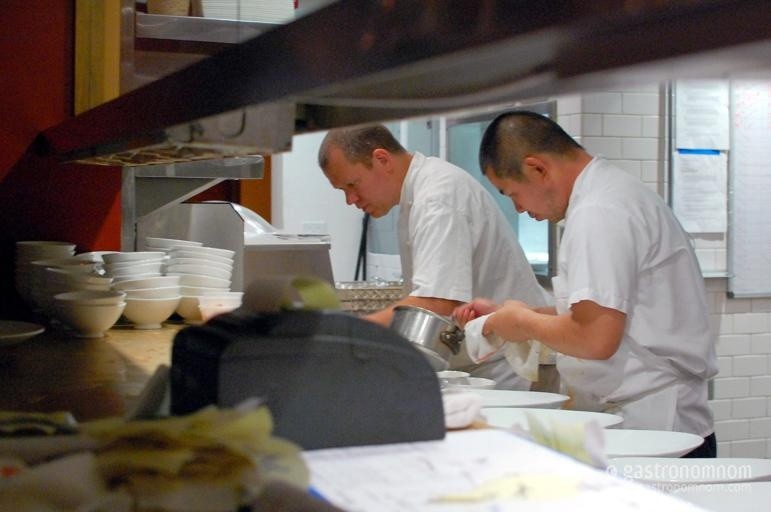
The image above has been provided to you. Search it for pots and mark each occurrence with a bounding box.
[387,304,471,376]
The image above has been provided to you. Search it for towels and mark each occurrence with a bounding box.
[465,310,542,382]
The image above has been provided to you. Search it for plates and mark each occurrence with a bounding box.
[283,427,703,511]
[0,319,45,347]
[429,361,771,511]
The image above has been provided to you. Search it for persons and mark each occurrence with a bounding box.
[451,109,721,456]
[317,122,558,392]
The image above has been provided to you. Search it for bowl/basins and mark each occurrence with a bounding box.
[12,236,246,331]
